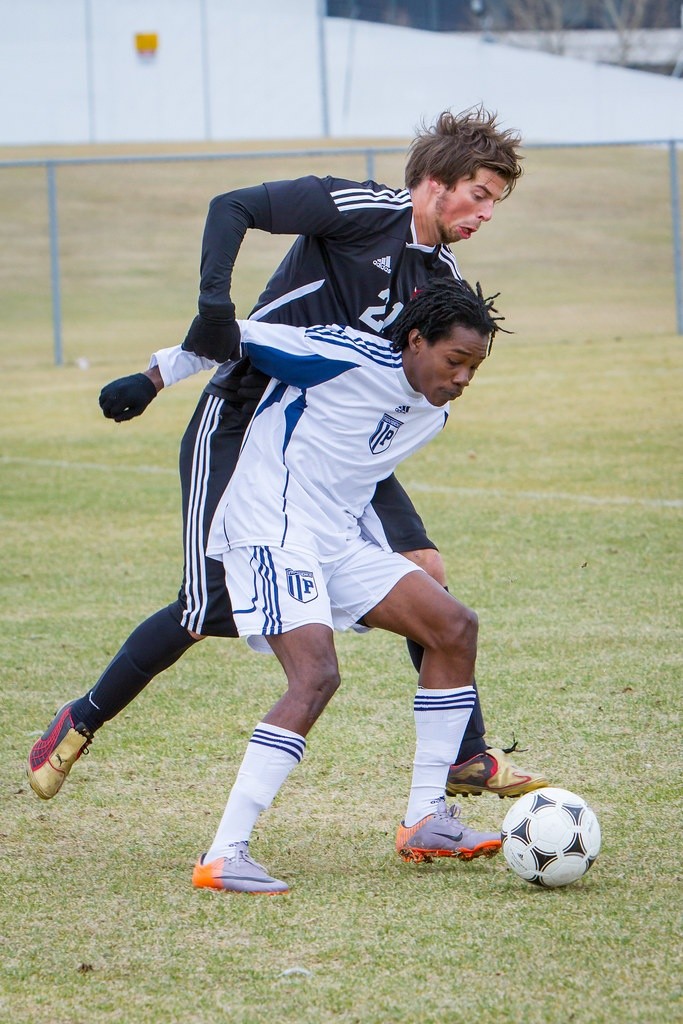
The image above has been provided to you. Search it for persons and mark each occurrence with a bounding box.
[23,105,549,799]
[98,273,502,897]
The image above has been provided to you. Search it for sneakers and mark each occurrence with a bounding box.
[394,812,502,864]
[192,851,290,895]
[25,700,95,800]
[444,748,550,799]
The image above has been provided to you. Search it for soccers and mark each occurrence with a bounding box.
[499,787,602,887]
